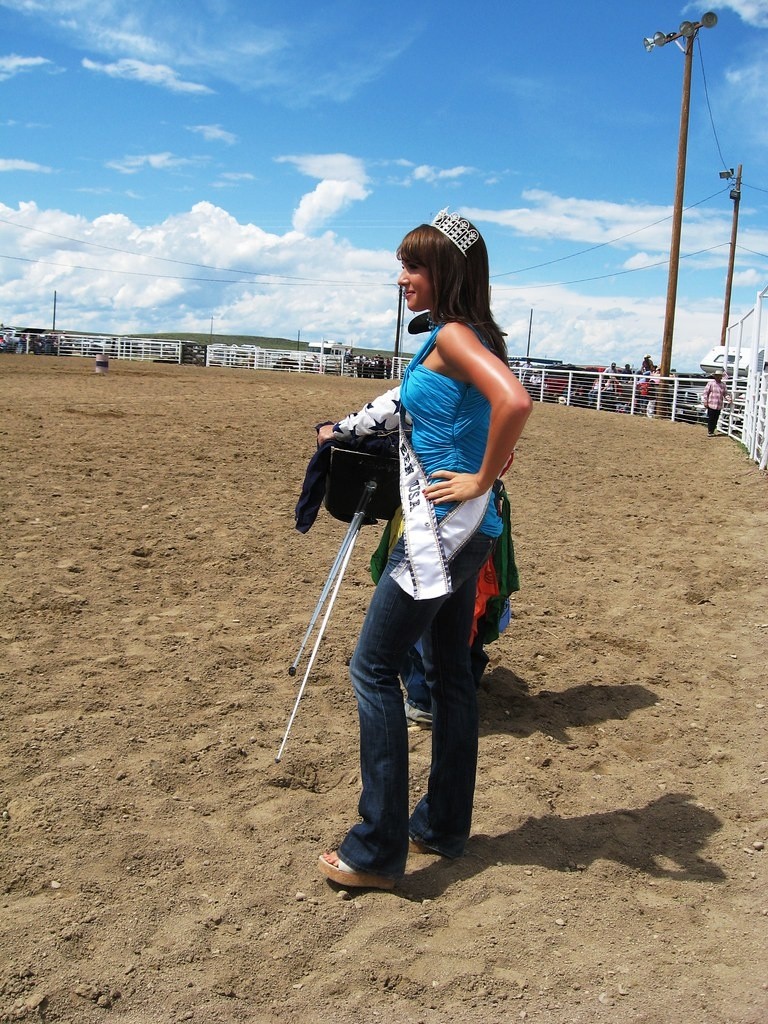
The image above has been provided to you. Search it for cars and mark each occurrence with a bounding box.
[675,379,748,425]
[0,328,72,355]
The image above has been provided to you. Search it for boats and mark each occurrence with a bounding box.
[700,347,768,377]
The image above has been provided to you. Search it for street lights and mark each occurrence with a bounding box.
[641,12,720,423]
[719,163,743,350]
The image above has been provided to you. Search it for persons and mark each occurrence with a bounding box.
[510,358,547,400]
[703,370,732,437]
[587,354,677,416]
[346,348,392,379]
[0,331,80,357]
[317,215,533,889]
[312,355,319,371]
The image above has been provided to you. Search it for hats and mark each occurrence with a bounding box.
[712,370,726,377]
[62,331,67,333]
[670,369,677,373]
[514,362,520,364]
[408,310,435,335]
[611,361,616,366]
[643,354,651,358]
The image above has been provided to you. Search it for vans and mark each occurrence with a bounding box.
[306,340,347,373]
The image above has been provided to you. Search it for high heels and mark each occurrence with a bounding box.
[318,851,398,890]
[408,837,437,854]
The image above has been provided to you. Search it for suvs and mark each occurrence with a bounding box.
[540,367,623,404]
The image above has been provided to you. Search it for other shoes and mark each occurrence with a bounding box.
[406,703,432,723]
[707,432,715,437]
